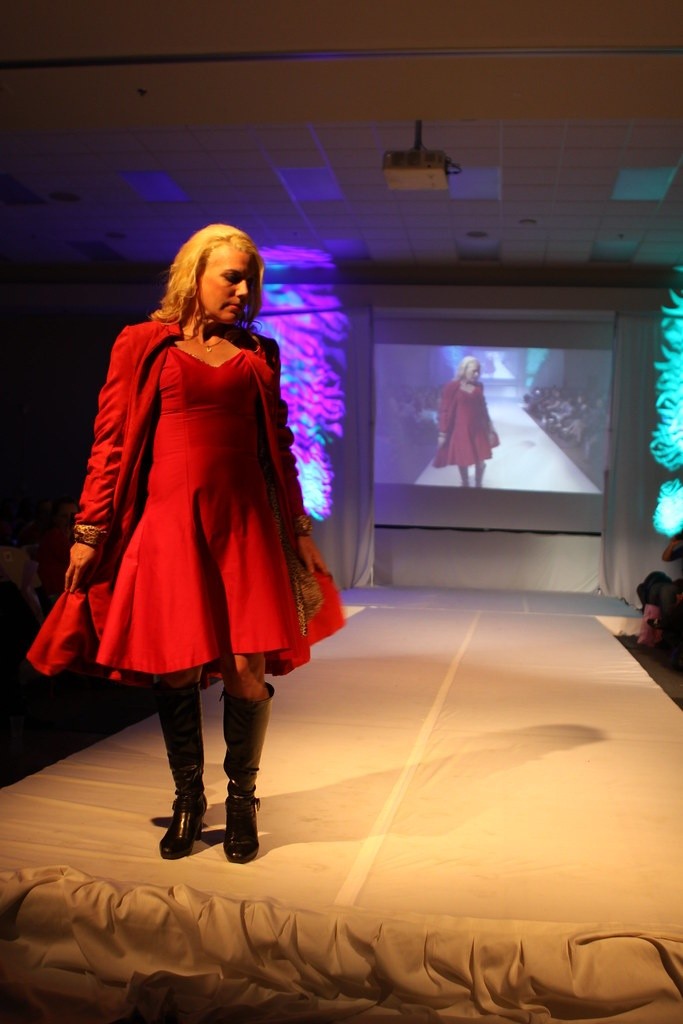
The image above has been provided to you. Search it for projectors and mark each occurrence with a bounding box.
[383,149,451,191]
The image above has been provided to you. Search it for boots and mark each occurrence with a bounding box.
[476,463,485,487]
[218,683,276,863]
[458,467,469,487]
[156,683,207,860]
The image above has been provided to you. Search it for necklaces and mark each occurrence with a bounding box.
[188,329,224,353]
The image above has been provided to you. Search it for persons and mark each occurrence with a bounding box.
[636,527,683,673]
[2,493,79,603]
[64,223,330,862]
[386,356,605,492]
